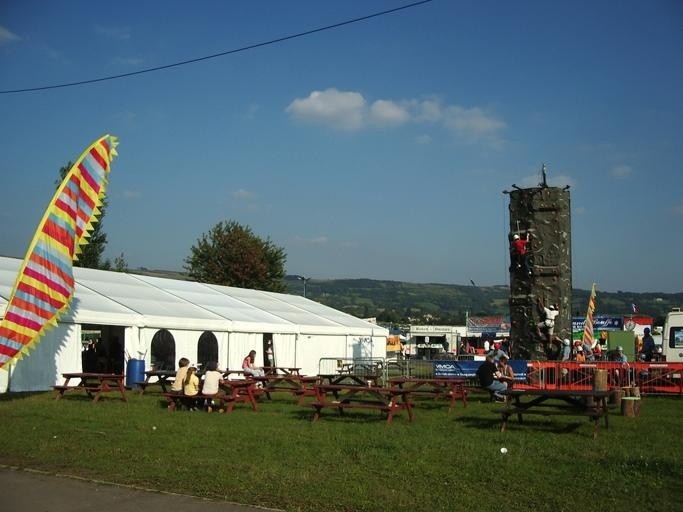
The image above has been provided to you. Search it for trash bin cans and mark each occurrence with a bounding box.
[591,369,608,405]
[126,360,145,388]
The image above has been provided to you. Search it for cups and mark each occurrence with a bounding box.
[366,380,371,388]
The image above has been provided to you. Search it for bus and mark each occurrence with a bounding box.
[662,306,682,382]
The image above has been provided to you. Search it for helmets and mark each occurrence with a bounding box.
[548,303,556,310]
[564,338,625,354]
[644,327,651,335]
[512,234,521,241]
[494,343,501,351]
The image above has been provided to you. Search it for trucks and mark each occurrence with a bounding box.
[572,329,608,353]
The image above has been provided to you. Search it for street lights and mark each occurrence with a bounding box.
[295,274,311,297]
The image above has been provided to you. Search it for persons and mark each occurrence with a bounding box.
[166,356,226,413]
[265,340,275,368]
[555,328,660,386]
[242,350,265,379]
[460,337,513,403]
[535,299,559,349]
[511,233,532,276]
